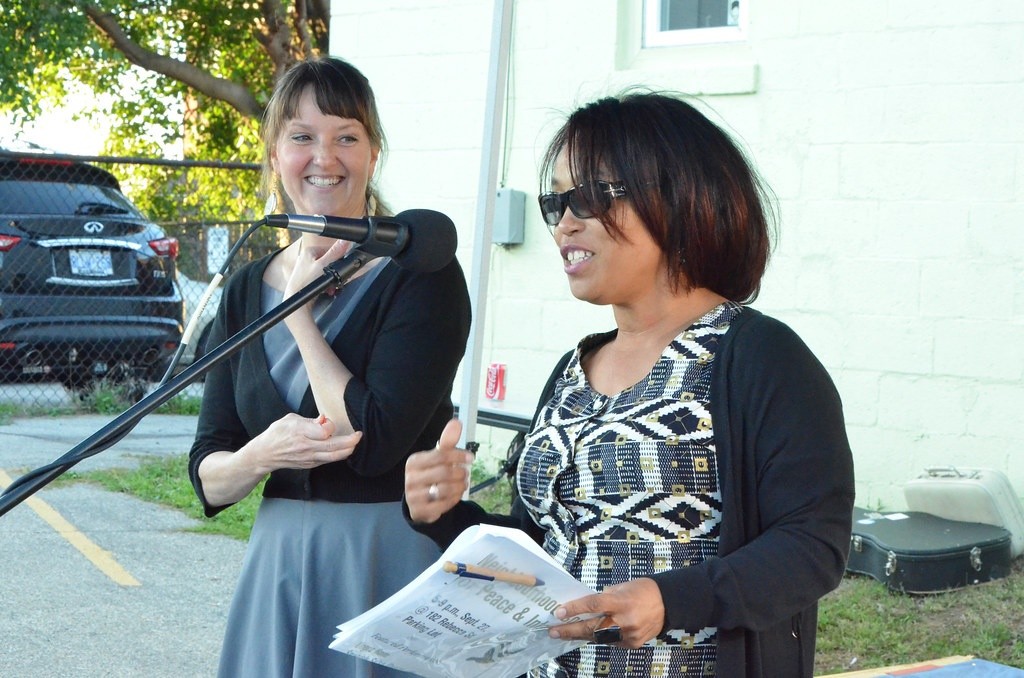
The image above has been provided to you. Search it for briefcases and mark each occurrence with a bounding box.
[904,464,1024,561]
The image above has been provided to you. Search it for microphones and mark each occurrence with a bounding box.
[265,209,458,272]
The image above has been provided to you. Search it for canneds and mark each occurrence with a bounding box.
[485,363,507,401]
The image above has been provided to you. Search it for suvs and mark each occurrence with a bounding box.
[0,150,182,409]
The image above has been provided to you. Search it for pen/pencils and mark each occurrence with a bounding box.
[443,562,545,588]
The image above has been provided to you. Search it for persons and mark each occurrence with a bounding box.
[188,58,472,678]
[401,96,855,678]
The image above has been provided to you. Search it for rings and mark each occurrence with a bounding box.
[429,483,439,500]
[593,616,622,644]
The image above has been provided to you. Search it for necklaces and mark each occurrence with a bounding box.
[298,239,302,255]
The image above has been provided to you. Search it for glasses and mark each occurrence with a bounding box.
[537,179,656,226]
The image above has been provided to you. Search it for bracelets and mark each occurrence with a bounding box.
[400,493,410,520]
